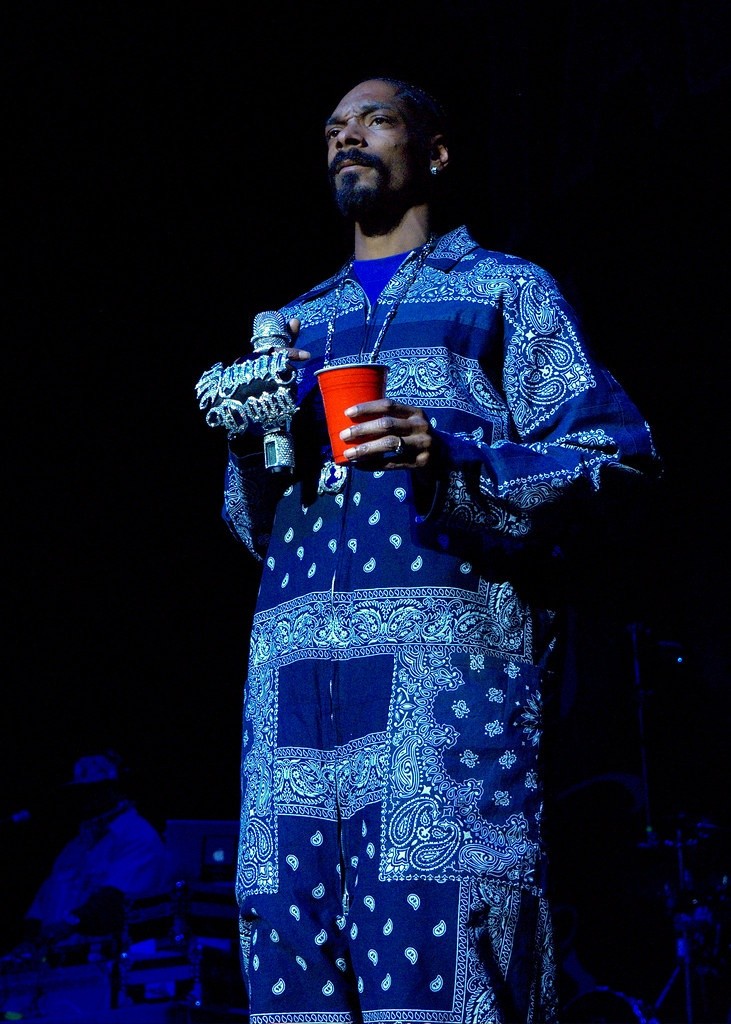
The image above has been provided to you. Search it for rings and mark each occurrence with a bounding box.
[396,437,403,454]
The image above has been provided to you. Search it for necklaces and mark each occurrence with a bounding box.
[316,238,435,496]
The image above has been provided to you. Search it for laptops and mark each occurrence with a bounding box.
[163,819,240,881]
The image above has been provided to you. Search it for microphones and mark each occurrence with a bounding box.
[12,809,31,823]
[251,310,295,477]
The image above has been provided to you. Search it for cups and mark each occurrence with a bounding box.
[313,363,390,465]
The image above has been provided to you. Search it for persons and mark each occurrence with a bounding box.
[194,76,663,1023]
[0,755,190,1024]
